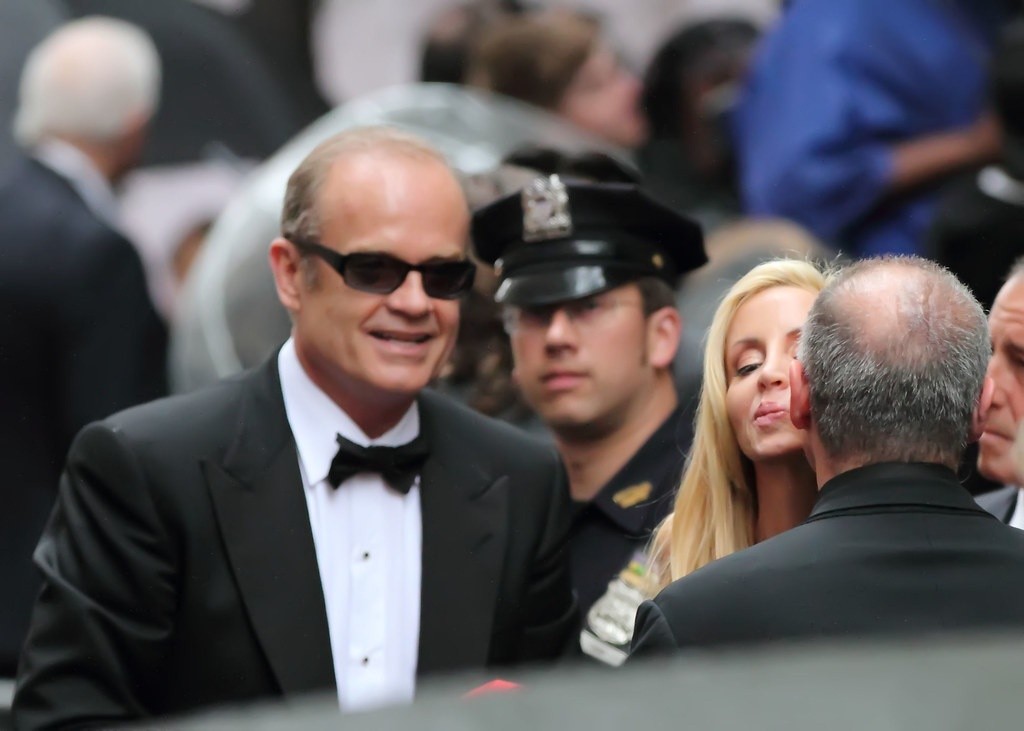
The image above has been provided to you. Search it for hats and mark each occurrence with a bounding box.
[471,184,708,305]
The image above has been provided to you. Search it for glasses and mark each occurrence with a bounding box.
[283,232,476,299]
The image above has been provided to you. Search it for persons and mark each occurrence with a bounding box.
[0,0,1024,731]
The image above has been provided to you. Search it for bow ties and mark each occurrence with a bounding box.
[326,433,419,494]
